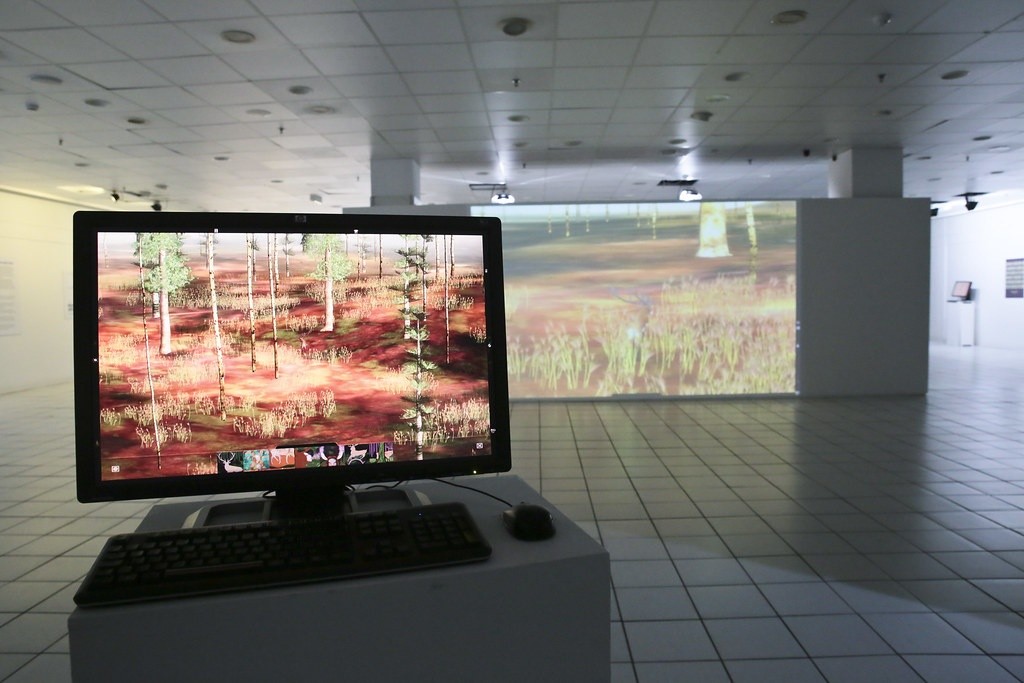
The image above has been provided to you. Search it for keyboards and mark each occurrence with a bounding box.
[73,500,493,607]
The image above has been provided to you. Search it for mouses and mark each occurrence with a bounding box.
[503,500,556,542]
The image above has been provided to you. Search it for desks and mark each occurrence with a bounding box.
[67,475,611,683]
[948,300,975,346]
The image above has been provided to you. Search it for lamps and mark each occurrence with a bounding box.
[151,200,161,211]
[491,183,515,204]
[111,190,119,202]
[965,193,978,210]
[679,182,703,202]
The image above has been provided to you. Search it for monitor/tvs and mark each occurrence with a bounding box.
[73,211,512,529]
[952,281,972,301]
